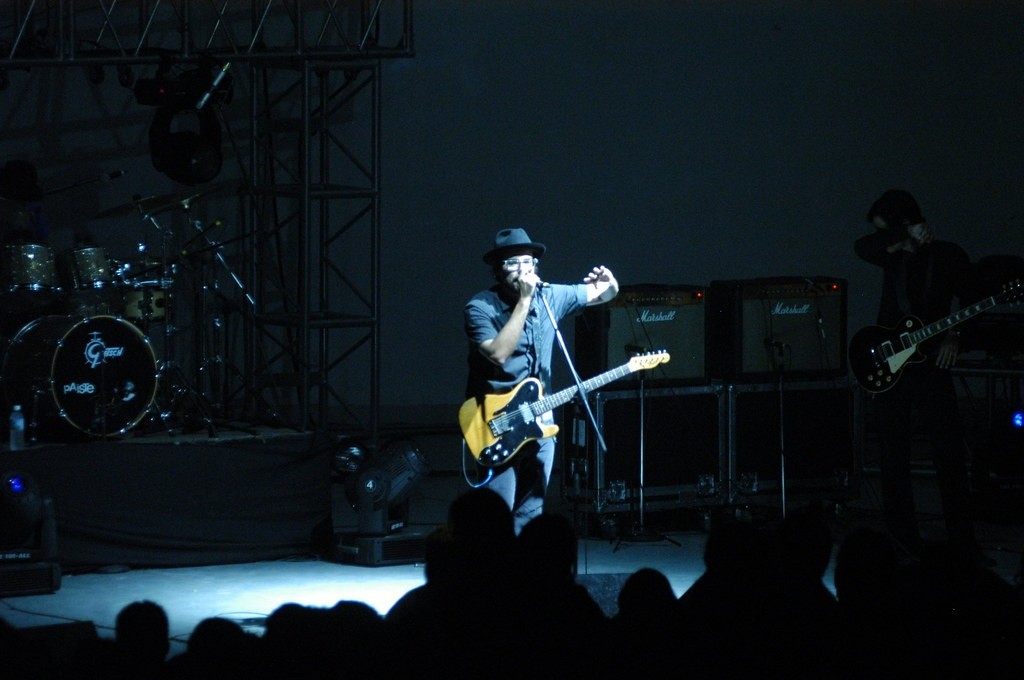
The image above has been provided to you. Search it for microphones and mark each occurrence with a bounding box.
[196,62,230,109]
[623,344,651,354]
[763,338,790,347]
[537,282,551,289]
[102,170,129,182]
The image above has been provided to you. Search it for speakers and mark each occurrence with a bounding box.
[707,274,848,385]
[574,283,710,388]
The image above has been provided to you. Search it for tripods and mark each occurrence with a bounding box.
[135,203,284,441]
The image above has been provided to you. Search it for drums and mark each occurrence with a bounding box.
[3,313,161,439]
[4,242,175,321]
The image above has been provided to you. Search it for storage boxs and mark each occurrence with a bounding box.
[569,386,730,533]
[728,384,862,517]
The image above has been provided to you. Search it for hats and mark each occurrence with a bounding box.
[483,228,546,266]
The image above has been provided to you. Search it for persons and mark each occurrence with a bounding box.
[461,227,620,537]
[113,487,1024,680]
[854,187,1000,570]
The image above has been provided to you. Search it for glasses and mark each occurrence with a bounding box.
[504,258,538,272]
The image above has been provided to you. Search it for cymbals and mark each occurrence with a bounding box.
[91,186,224,217]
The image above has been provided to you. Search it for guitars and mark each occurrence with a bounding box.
[846,276,1023,397]
[458,348,671,468]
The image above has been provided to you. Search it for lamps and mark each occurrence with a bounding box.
[331,440,432,539]
[0,465,60,547]
[150,100,228,182]
